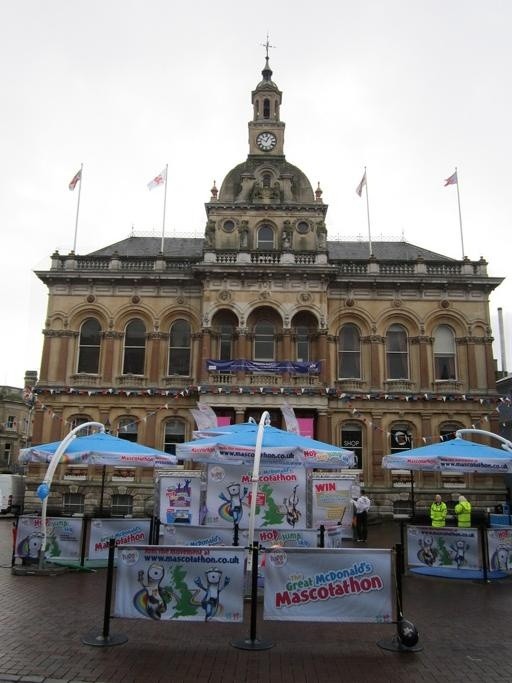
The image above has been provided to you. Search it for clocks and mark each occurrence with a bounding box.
[255,131,277,152]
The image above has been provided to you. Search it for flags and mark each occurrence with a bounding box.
[442,167,460,190]
[66,163,84,192]
[353,166,369,198]
[146,164,169,191]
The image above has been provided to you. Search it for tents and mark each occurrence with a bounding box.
[16,411,354,590]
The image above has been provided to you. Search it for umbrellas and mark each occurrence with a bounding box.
[380,429,512,474]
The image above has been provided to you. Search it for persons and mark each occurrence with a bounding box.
[430,494,448,527]
[351,490,371,543]
[454,495,472,528]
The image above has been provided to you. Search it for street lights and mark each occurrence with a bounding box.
[405,422,417,521]
[99,416,111,514]
